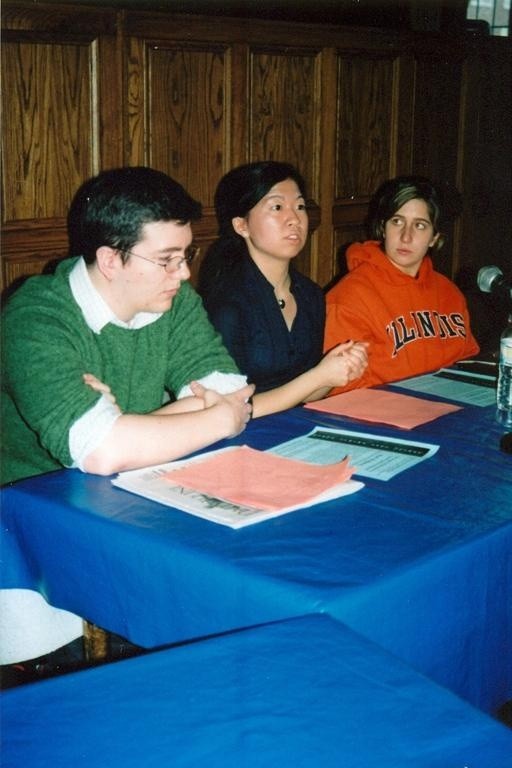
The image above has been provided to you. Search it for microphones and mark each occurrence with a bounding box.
[476,265,512,302]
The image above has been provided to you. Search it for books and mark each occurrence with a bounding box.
[106,445,365,530]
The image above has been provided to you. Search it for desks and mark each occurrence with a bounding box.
[0,358,512,714]
[0,614,512,768]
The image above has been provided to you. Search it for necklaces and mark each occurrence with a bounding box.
[270,282,291,309]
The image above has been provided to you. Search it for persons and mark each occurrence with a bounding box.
[0,167,256,663]
[322,177,479,398]
[206,160,369,420]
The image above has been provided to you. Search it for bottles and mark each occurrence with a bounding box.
[497,310,512,411]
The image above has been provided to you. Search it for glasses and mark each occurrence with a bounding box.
[109,243,202,272]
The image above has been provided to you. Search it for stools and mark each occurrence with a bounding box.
[0,589,86,692]
